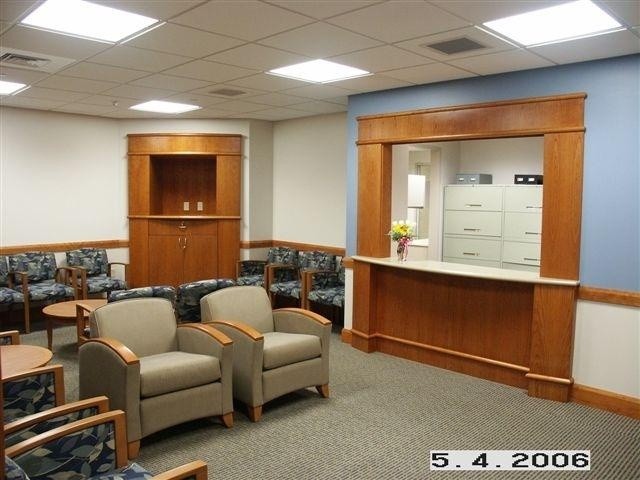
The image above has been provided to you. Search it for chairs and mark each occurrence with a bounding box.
[79,298,233,459]
[268,250,330,308]
[9,253,78,333]
[1,364,109,451]
[307,255,345,311]
[1,409,208,479]
[0,255,30,333]
[67,248,130,301]
[201,285,332,423]
[236,247,294,289]
[76,285,176,347]
[177,279,234,325]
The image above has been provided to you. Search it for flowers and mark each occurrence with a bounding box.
[384,225,415,242]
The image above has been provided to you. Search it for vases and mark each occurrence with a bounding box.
[397,238,409,261]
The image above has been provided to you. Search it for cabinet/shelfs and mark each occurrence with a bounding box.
[442,185,542,274]
[149,219,218,286]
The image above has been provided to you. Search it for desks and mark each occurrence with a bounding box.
[44,298,108,350]
[0,345,52,378]
